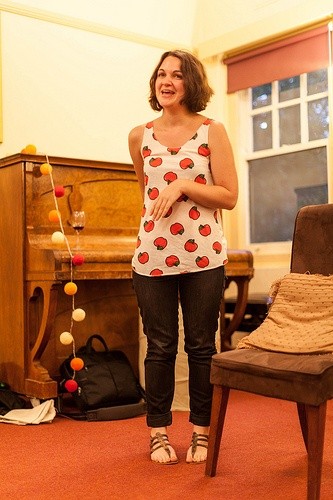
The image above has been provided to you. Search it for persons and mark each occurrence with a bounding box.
[126,50,238,465]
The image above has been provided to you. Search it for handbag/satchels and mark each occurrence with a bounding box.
[0,381,28,416]
[55,334,147,421]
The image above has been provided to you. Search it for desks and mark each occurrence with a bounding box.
[224,295,271,334]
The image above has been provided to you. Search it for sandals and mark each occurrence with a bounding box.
[150,432,179,465]
[188,432,209,464]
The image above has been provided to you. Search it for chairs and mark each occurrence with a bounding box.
[206,203,333,500]
